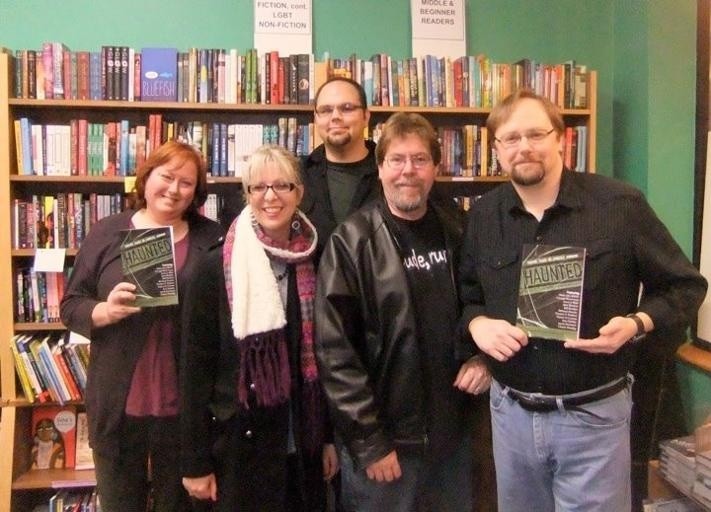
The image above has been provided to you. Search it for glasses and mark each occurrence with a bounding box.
[382,153,433,167]
[247,182,298,193]
[493,127,557,145]
[313,104,367,116]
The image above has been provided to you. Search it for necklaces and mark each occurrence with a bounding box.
[271,260,291,286]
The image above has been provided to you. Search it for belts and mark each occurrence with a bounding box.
[488,373,630,415]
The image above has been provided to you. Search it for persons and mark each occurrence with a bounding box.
[314,114,491,510]
[298,76,384,248]
[178,144,340,510]
[59,141,226,512]
[458,89,697,512]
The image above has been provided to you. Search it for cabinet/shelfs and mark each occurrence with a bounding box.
[0,44,597,512]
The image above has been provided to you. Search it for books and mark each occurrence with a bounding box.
[641,420,711,512]
[142,482,162,510]
[13,190,134,252]
[115,225,181,309]
[430,118,509,178]
[75,411,95,472]
[567,125,587,173]
[10,332,91,407]
[27,405,76,473]
[513,240,588,344]
[199,192,226,226]
[33,489,102,512]
[364,119,383,154]
[16,264,76,323]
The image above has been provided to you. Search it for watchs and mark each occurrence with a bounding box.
[626,312,649,346]
